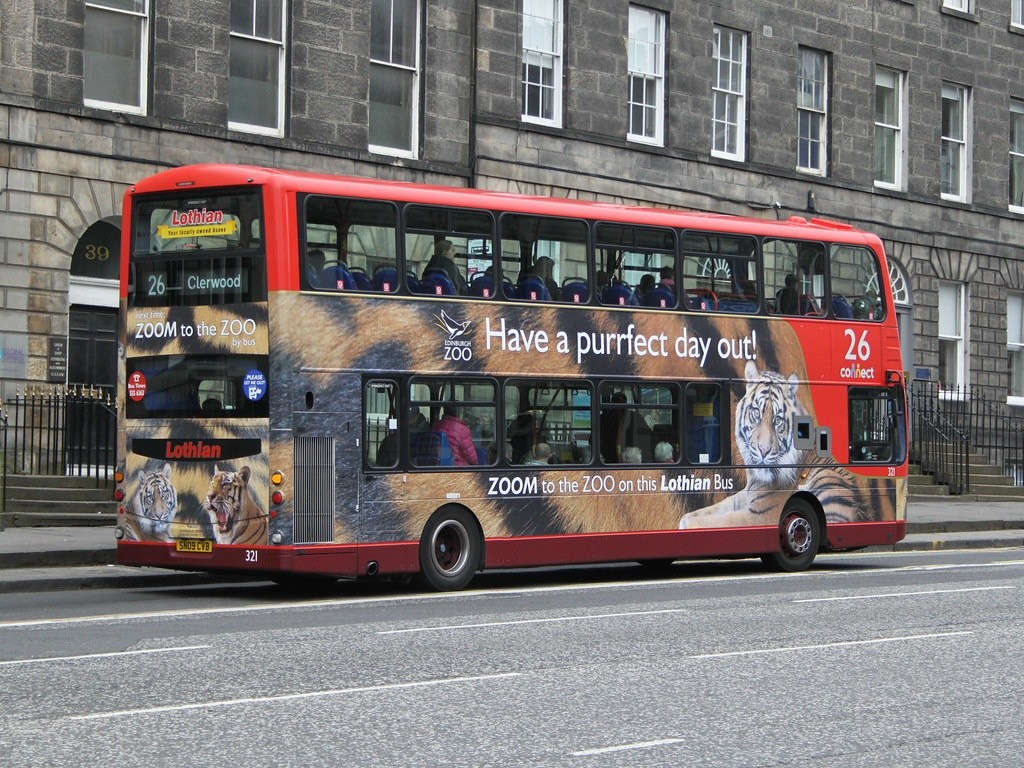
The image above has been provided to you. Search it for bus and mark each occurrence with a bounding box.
[111,162,914,595]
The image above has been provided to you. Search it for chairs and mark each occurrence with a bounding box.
[375,433,490,474]
[303,264,878,329]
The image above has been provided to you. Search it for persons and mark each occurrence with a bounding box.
[201,399,222,409]
[308,249,326,271]
[512,411,546,462]
[488,442,511,463]
[660,267,692,308]
[622,447,641,464]
[641,274,655,291]
[857,290,877,319]
[535,257,565,301]
[409,406,427,432]
[485,266,504,277]
[597,270,608,287]
[588,392,652,462]
[424,241,470,295]
[774,275,805,315]
[431,405,478,465]
[655,441,674,463]
[524,443,552,465]
